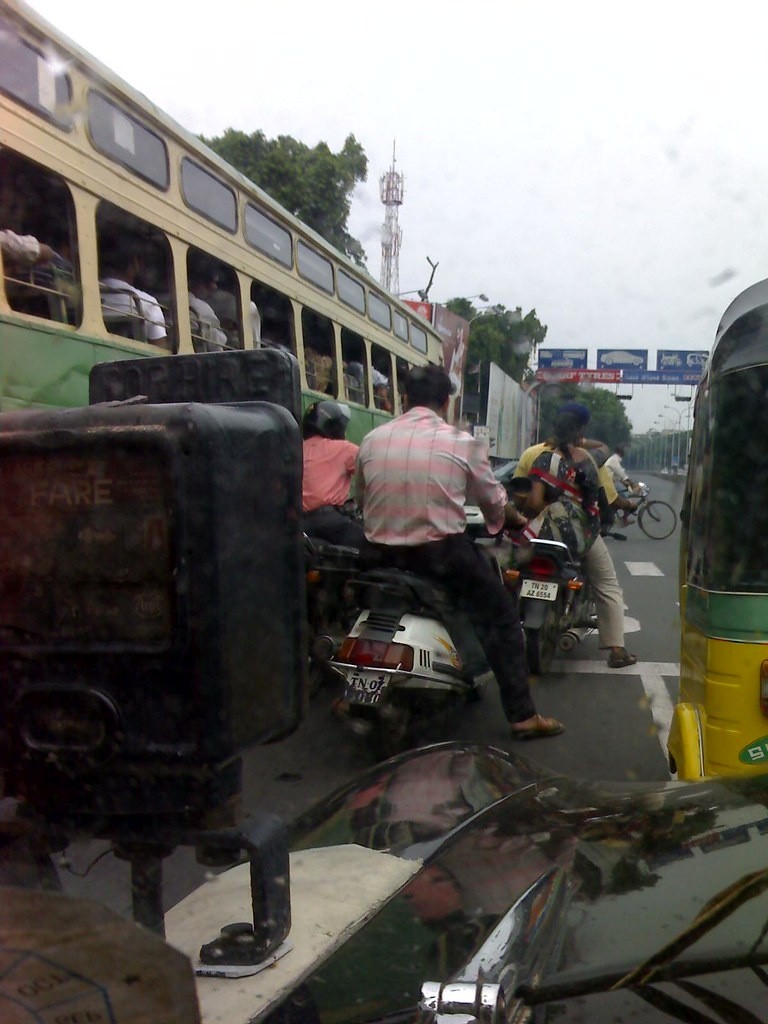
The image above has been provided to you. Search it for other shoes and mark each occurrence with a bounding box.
[510,714,564,741]
[624,520,635,526]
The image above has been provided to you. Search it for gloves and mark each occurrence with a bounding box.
[622,499,638,513]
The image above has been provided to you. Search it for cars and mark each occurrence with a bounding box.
[461,458,519,549]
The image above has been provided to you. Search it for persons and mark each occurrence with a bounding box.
[448,321,465,392]
[353,367,566,740]
[0,187,642,667]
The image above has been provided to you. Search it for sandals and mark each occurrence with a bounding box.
[607,647,637,668]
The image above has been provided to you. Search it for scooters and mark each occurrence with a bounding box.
[311,501,525,761]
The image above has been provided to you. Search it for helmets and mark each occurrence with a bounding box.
[303,401,352,440]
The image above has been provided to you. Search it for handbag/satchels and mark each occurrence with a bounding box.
[522,497,602,557]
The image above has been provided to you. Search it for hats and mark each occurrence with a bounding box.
[557,403,589,426]
[397,366,456,402]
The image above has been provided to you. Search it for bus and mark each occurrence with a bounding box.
[0,0,457,503]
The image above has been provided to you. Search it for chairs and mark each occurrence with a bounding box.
[164,305,207,353]
[344,369,363,404]
[100,286,148,342]
[5,259,69,324]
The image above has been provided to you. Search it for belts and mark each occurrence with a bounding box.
[302,506,338,519]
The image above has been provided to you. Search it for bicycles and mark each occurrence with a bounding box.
[600,482,678,538]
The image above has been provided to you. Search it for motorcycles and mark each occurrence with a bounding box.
[666,275,768,781]
[301,494,372,639]
[500,470,639,677]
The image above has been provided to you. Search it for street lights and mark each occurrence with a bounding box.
[665,405,695,469]
[659,415,683,468]
